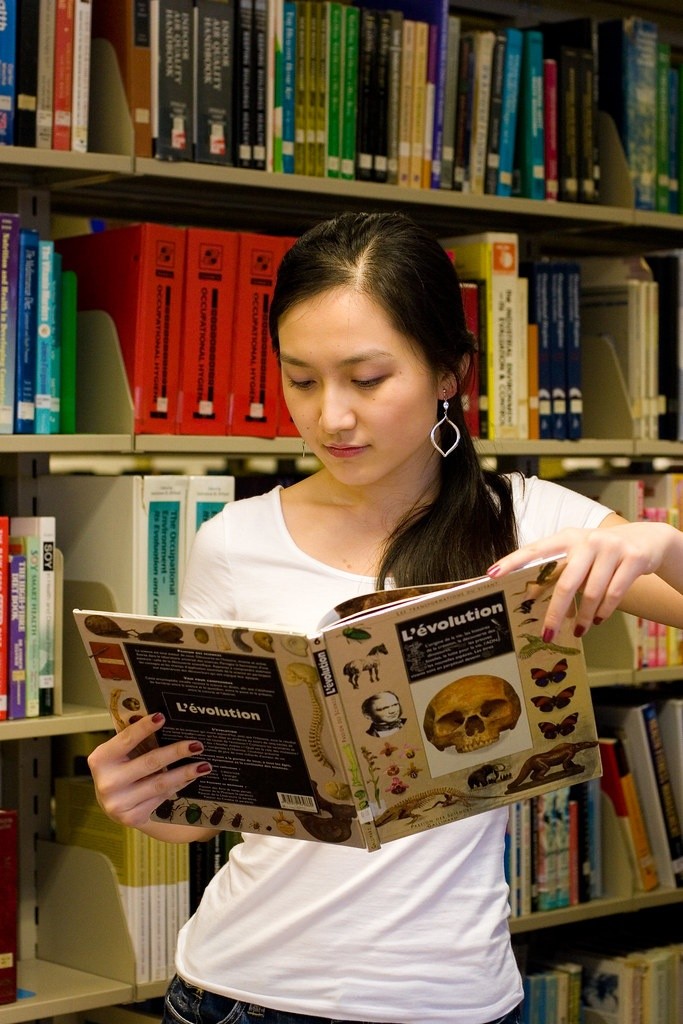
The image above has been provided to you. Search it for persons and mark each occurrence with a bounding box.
[360,690,408,741]
[86,206,683,1024]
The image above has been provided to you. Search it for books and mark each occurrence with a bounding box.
[0,0,683,1024]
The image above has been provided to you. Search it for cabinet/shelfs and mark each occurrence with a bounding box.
[0,0,683,1023]
